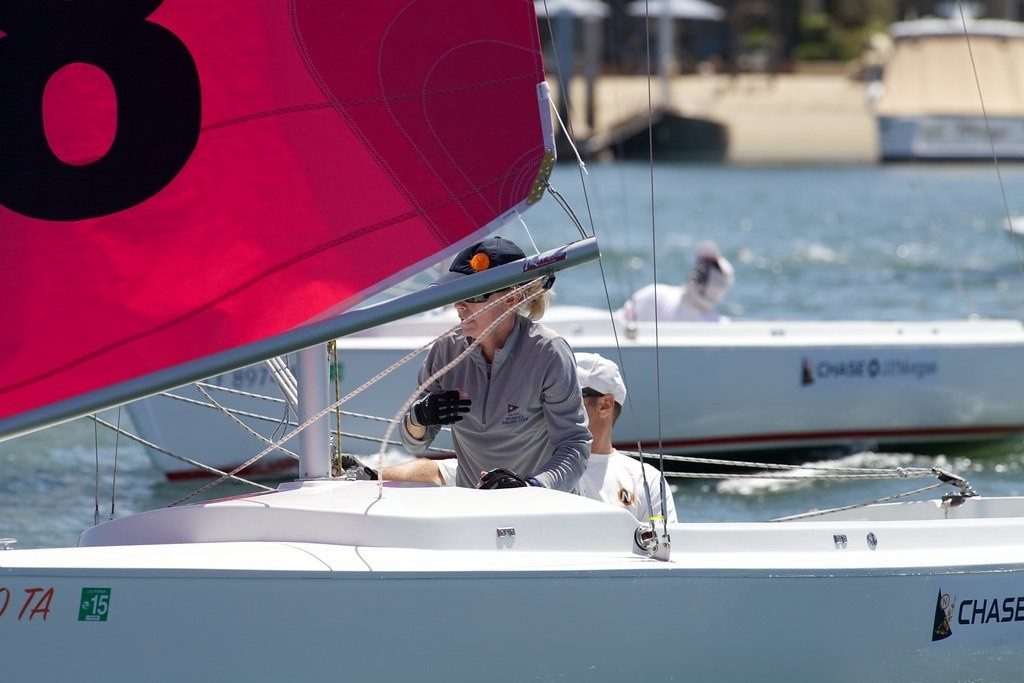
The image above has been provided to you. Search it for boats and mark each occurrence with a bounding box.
[125,303,1024,484]
[878,17,1023,161]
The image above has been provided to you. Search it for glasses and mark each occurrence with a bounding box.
[465,287,511,303]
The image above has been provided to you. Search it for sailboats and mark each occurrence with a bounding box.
[0,0,1024,683]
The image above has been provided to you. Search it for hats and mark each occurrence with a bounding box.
[574,352,627,406]
[428,236,526,287]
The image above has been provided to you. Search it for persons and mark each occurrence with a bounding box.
[373,351,678,523]
[398,237,592,498]
[617,245,733,323]
